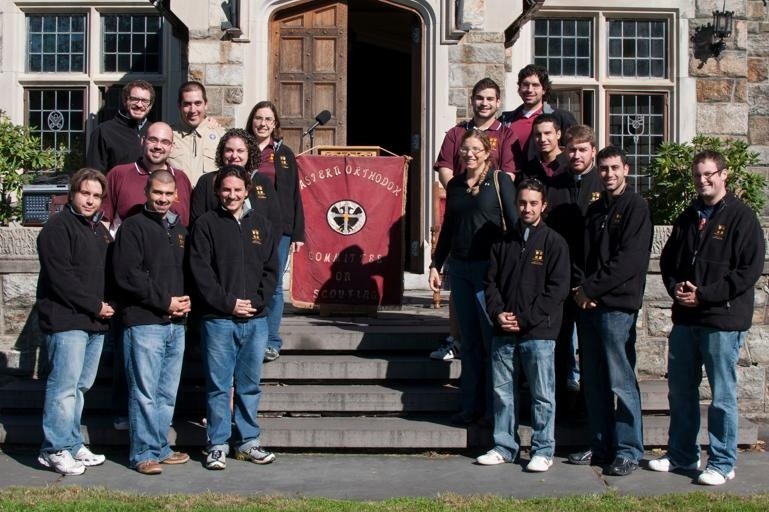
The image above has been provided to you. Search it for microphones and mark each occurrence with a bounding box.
[302,110,331,136]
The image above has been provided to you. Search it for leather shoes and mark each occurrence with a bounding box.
[602,453,640,475]
[569,448,609,466]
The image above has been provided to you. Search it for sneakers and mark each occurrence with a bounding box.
[263,345,279,362]
[475,448,508,465]
[113,417,130,431]
[649,450,703,472]
[137,449,226,474]
[697,462,736,487]
[234,441,277,465]
[38,449,87,476]
[525,454,554,472]
[74,445,106,466]
[430,335,464,361]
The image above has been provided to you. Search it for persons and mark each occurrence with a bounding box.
[187,166,280,470]
[430,129,520,430]
[514,115,578,196]
[648,150,766,486]
[568,146,654,475]
[246,101,305,362]
[87,82,156,175]
[543,125,605,400]
[475,179,569,472]
[498,67,578,194]
[167,82,226,189]
[191,128,282,248]
[431,78,522,360]
[36,168,115,476]
[101,122,194,240]
[115,169,191,474]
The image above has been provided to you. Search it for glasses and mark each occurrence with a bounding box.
[147,137,174,145]
[693,171,722,179]
[459,146,486,154]
[129,96,152,107]
